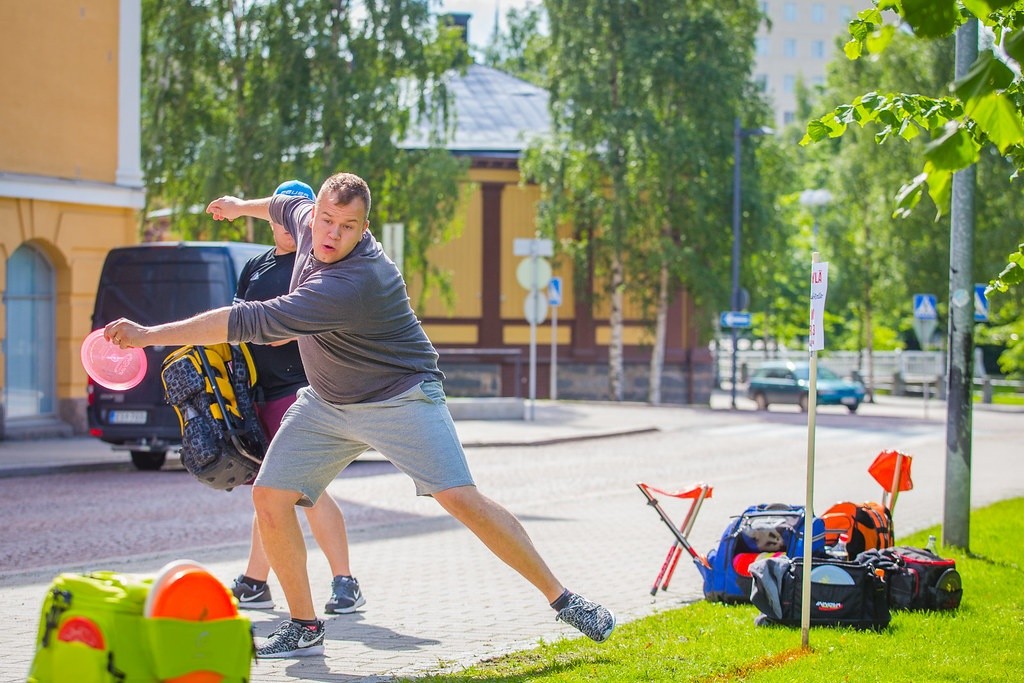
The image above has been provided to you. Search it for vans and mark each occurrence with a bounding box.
[86,241,277,470]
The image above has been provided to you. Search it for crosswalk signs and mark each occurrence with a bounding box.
[912,293,938,320]
[549,279,562,305]
[974,285,989,321]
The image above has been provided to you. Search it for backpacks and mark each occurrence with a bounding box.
[821,499,895,559]
[159,338,271,492]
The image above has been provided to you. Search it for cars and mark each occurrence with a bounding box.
[747,362,865,416]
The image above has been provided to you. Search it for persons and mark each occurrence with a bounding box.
[230,181,366,615]
[103,173,615,658]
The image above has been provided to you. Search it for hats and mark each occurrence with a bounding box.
[273,180,316,205]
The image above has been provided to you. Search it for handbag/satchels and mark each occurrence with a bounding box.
[692,504,827,604]
[24,559,253,682]
[747,555,891,633]
[855,546,963,615]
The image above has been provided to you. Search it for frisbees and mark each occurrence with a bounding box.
[79,327,148,393]
[150,568,239,622]
[144,558,210,618]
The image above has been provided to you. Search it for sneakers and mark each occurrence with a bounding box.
[555,593,617,645]
[324,574,366,614]
[254,619,326,658]
[225,574,274,609]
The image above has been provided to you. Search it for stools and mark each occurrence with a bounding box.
[635,484,713,598]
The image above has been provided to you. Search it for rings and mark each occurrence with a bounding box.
[115,337,121,342]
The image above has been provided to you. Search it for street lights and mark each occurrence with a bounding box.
[730,126,772,406]
[799,188,836,252]
